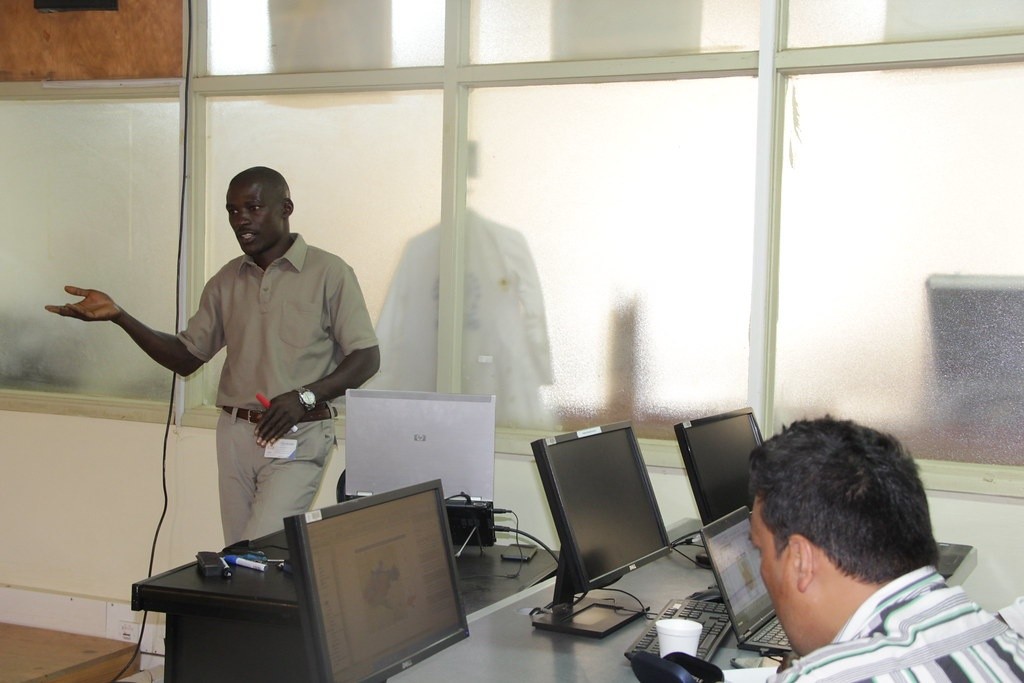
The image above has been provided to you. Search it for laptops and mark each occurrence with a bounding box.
[699,506,796,656]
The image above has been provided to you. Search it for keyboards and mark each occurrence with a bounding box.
[625,599,732,682]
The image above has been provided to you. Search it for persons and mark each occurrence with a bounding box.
[748,413,1024,682]
[45,166,382,551]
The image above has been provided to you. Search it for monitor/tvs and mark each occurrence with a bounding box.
[283,478,469,682]
[345,388,497,504]
[673,407,766,525]
[530,420,672,593]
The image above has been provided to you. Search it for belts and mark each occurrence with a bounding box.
[221,405,339,423]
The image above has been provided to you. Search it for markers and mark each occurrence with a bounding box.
[255,393,299,432]
[217,553,233,578]
[223,555,267,572]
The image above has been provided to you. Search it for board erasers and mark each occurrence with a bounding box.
[195,551,225,577]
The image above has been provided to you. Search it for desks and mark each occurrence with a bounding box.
[1,620,141,683]
[382,521,974,683]
[130,525,561,683]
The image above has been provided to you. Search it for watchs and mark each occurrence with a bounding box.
[294,387,317,413]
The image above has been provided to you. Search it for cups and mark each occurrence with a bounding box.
[655,618,704,659]
